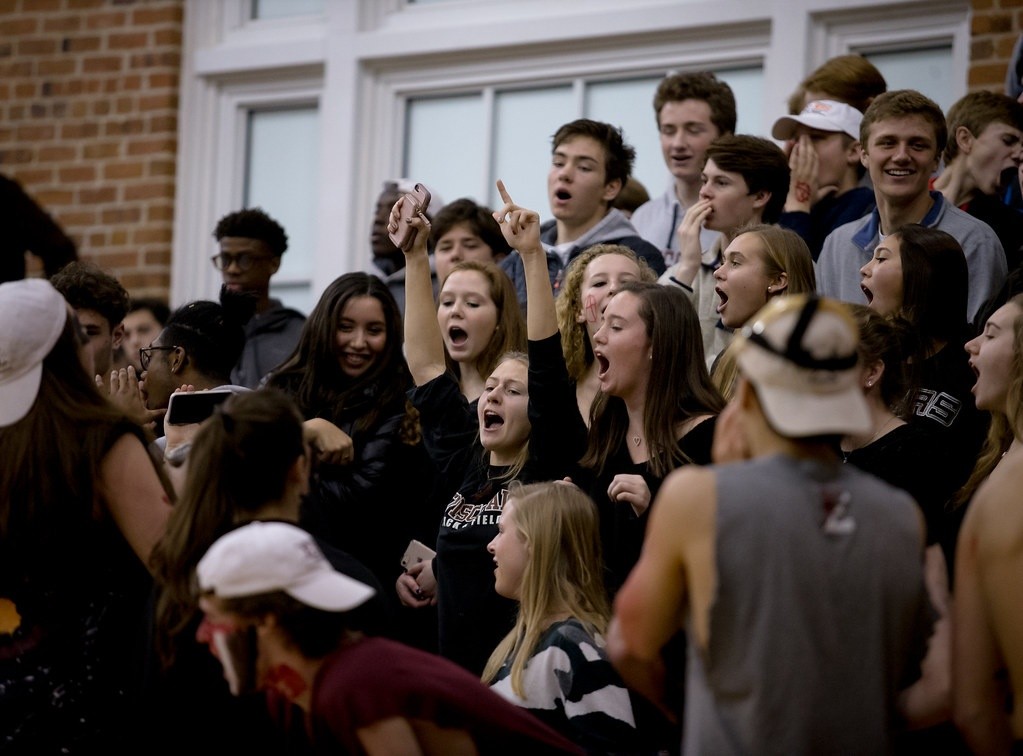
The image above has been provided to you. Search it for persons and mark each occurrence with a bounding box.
[0,53,1023,756]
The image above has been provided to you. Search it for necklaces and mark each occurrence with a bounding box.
[630,425,644,447]
[843,416,896,463]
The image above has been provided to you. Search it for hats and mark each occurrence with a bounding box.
[0,278,68,426]
[772,99,865,141]
[734,293,874,438]
[195,521,375,612]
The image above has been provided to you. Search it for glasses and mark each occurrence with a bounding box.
[139,344,178,371]
[211,249,275,271]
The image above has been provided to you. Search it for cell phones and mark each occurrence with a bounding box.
[401,539,437,575]
[167,390,231,426]
[388,193,419,248]
[401,184,431,251]
